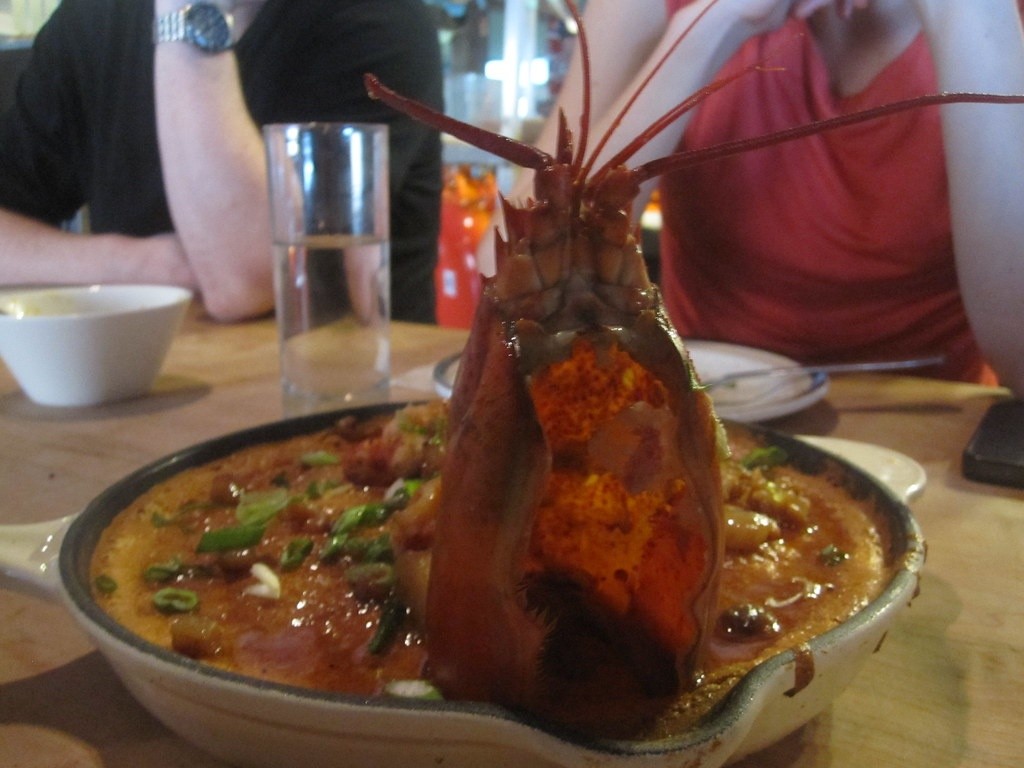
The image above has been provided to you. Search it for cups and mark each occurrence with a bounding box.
[265,122,396,420]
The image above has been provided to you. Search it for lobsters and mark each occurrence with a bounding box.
[362,0,1023,746]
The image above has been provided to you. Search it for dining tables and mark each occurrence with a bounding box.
[0,283,1024,768]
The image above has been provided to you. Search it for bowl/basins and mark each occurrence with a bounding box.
[0,283,194,407]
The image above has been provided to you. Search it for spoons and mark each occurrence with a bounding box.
[703,353,946,393]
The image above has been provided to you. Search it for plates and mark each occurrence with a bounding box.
[435,331,827,427]
[1,392,928,768]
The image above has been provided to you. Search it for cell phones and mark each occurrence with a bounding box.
[962,399,1023,487]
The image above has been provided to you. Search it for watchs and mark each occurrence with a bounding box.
[151,1,239,54]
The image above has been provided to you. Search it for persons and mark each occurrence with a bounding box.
[469,0,1024,405]
[0,0,448,325]
